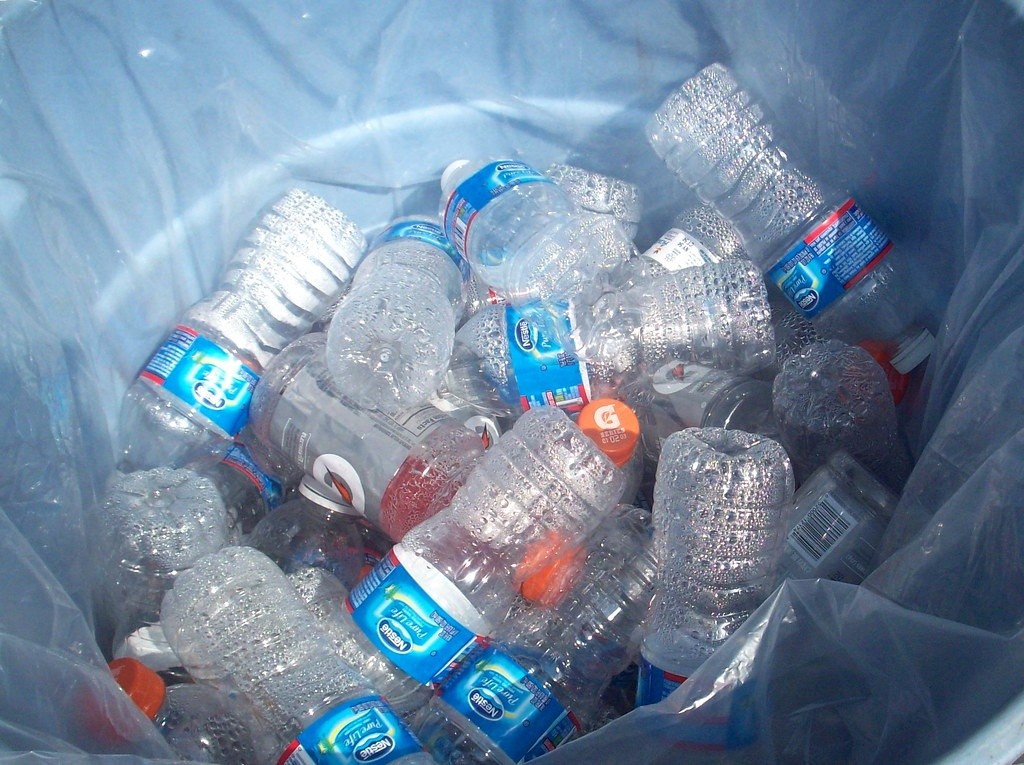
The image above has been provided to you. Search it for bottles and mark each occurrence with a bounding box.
[63,54,945,761]
